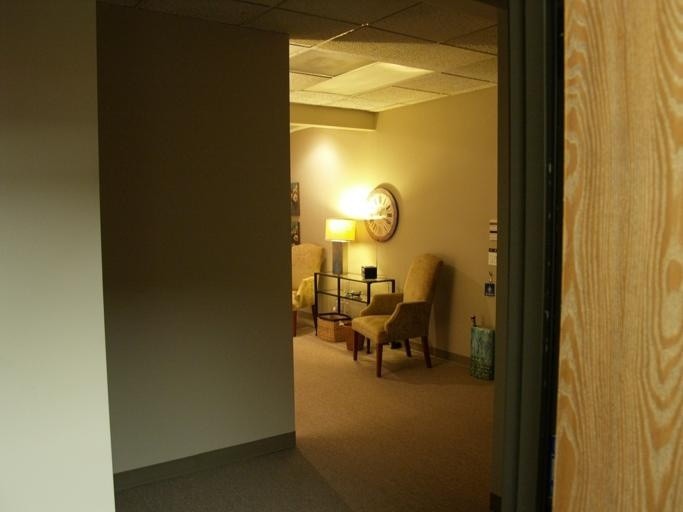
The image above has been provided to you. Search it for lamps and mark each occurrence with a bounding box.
[325,216,356,273]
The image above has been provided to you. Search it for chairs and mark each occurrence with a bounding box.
[291,242,323,337]
[350,254,444,378]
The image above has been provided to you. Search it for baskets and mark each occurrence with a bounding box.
[317,313,352,343]
[342,321,364,351]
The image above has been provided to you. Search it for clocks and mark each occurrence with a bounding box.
[364,186,399,243]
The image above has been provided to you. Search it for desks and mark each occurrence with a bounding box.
[313,269,398,356]
